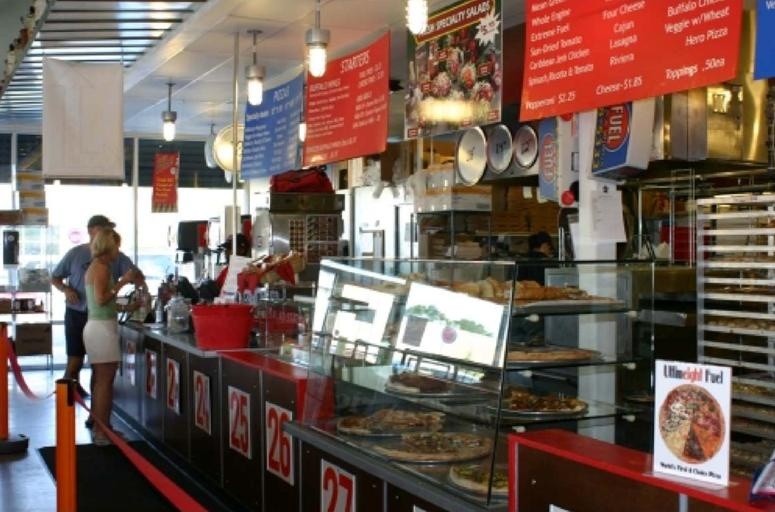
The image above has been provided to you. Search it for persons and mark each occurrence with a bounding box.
[48,213,147,401]
[211,232,251,289]
[82,225,141,445]
[511,228,560,286]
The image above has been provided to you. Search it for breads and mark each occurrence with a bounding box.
[388,272,615,304]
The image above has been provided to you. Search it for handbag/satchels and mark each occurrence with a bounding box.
[118,289,156,324]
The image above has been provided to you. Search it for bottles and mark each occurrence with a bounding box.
[168,297,191,336]
[153,298,165,324]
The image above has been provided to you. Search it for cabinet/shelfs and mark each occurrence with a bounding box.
[283,256,658,512]
[0,224,54,375]
[695,196,775,485]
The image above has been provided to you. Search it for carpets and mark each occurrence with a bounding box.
[38,439,227,512]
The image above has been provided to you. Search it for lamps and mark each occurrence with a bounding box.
[405,0,428,34]
[305,0,330,77]
[245,29,266,105]
[162,83,177,142]
[204,124,217,168]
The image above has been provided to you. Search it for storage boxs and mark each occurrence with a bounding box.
[12,322,52,355]
[0,173,48,224]
[17,268,50,291]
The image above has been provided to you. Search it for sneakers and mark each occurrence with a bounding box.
[91,424,124,441]
[92,431,130,446]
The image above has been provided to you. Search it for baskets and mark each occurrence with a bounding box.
[244,252,305,286]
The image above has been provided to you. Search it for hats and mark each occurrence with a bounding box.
[88,215,116,228]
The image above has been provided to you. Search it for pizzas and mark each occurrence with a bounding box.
[506,345,593,360]
[505,388,587,414]
[371,431,494,462]
[658,384,725,464]
[336,408,448,436]
[449,462,509,497]
[386,369,451,395]
